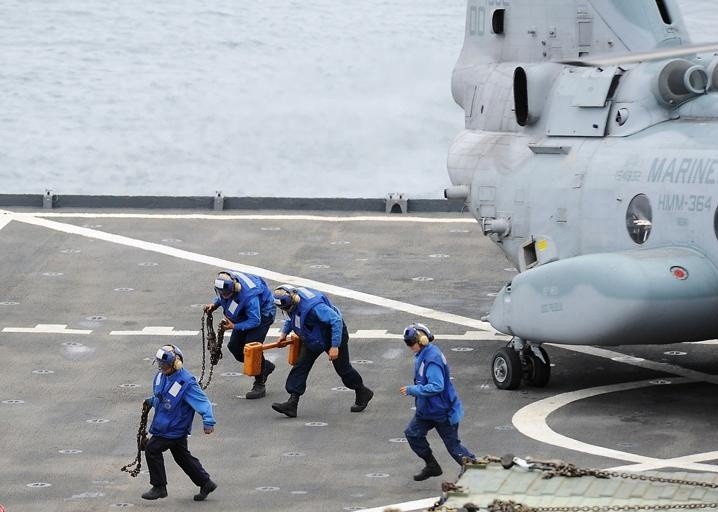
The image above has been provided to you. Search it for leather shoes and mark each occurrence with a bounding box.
[142,485,168,500]
[193,480,217,501]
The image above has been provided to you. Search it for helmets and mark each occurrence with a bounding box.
[214,270,241,296]
[273,285,298,310]
[155,344,183,370]
[403,323,434,346]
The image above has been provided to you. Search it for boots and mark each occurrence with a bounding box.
[413,455,443,482]
[263,360,275,382]
[272,394,300,418]
[350,384,374,412]
[245,378,266,400]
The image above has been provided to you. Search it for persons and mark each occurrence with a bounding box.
[270,284,374,418]
[201,269,277,399]
[140,342,217,502]
[397,321,476,482]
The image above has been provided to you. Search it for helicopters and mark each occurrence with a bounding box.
[440,0,717,391]
